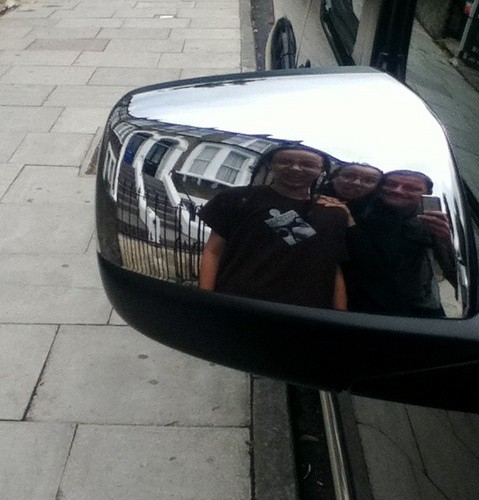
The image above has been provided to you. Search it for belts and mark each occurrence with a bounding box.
[267,157,323,171]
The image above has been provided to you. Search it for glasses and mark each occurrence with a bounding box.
[333,171,378,185]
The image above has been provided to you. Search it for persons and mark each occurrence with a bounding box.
[372,168,460,320]
[312,163,409,320]
[195,145,349,311]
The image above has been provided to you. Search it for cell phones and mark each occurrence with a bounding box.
[421,195,441,214]
